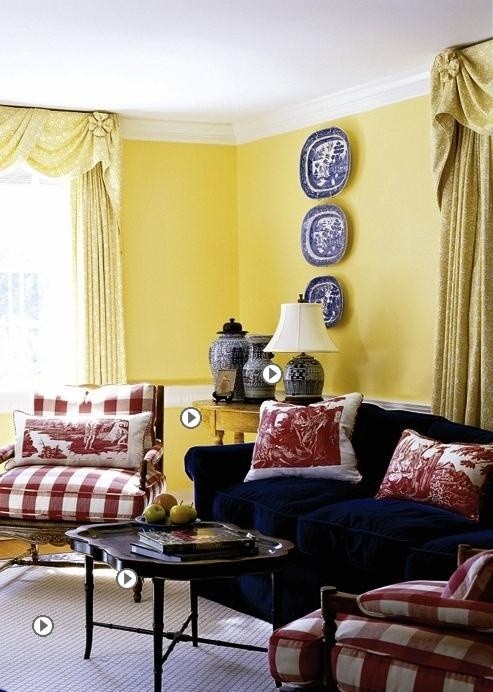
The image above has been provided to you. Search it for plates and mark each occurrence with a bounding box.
[300,203,349,268]
[135,514,201,529]
[304,276,343,329]
[299,127,351,202]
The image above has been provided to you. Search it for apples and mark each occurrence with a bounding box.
[145,505,166,524]
[186,502,196,519]
[170,500,190,524]
[154,494,177,515]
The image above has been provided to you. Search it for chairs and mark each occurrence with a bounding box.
[0,382,166,602]
[270,544,492,692]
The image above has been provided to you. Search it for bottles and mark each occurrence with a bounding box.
[208,317,276,401]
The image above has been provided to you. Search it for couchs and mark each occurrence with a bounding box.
[185,403,492,628]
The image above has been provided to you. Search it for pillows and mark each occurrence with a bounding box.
[436,549,493,601]
[5,410,152,474]
[244,393,493,524]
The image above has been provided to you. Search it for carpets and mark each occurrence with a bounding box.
[1,550,275,690]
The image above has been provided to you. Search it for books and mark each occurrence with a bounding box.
[129,542,259,563]
[136,526,254,554]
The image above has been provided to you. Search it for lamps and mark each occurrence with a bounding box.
[262,292,340,406]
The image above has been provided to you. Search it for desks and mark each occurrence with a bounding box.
[193,400,260,445]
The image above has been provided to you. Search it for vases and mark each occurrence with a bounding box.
[241,335,278,404]
